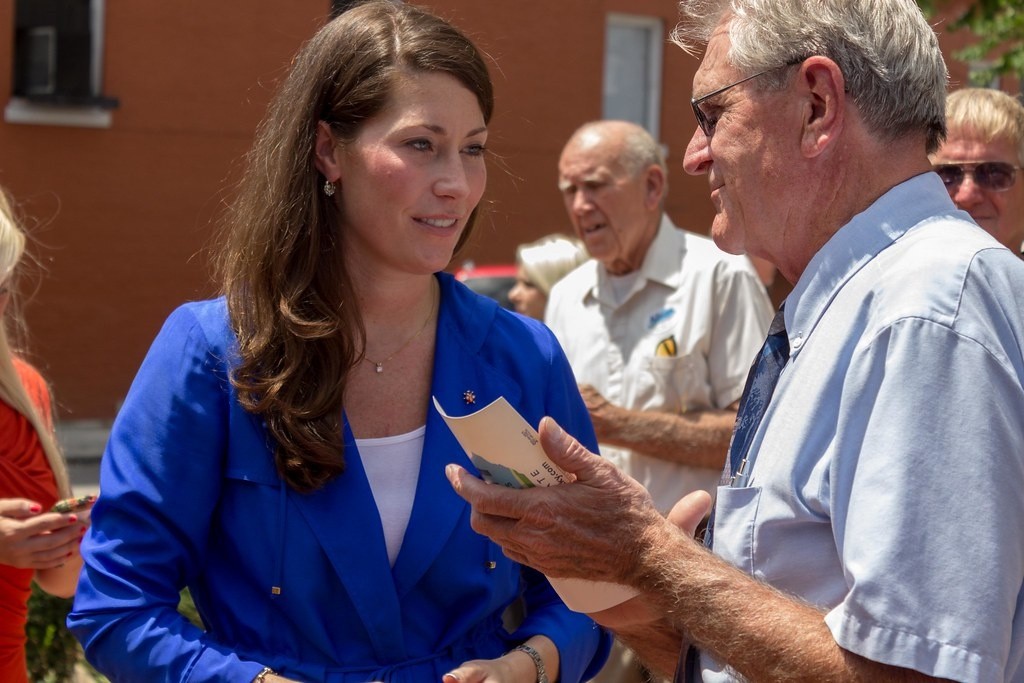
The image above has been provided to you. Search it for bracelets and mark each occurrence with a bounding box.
[502,644,547,683]
[257,667,278,683]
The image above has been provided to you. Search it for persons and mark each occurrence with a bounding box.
[445,0,1024,683]
[0,189,98,683]
[68,0,614,683]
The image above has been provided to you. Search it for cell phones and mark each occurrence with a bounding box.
[49,495,98,513]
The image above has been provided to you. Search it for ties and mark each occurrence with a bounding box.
[672,300,790,683]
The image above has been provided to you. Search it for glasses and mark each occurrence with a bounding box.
[931,161,1021,192]
[690,56,850,137]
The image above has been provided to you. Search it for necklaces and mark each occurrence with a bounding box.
[351,281,436,373]
